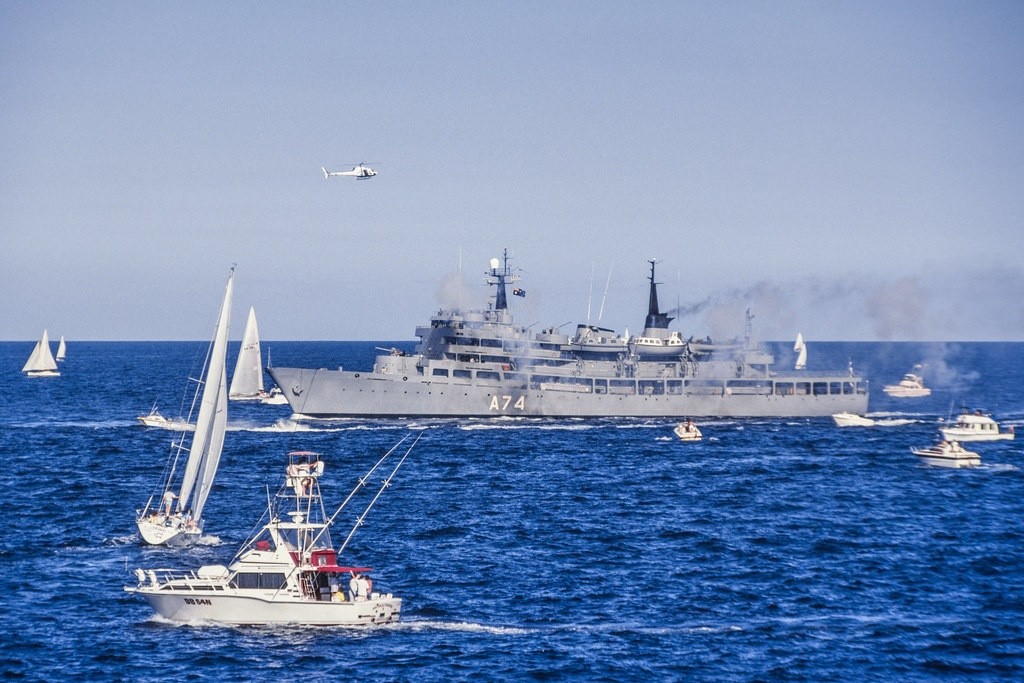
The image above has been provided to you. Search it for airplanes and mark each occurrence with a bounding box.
[321,160,377,183]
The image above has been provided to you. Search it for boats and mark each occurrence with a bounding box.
[831,409,875,427]
[671,416,704,441]
[627,331,687,358]
[935,399,1016,442]
[566,262,628,354]
[135,400,197,434]
[119,432,425,629]
[882,364,932,398]
[261,244,870,426]
[910,440,982,469]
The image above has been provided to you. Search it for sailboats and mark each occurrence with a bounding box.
[55,335,69,359]
[21,329,63,378]
[226,306,290,405]
[133,261,239,549]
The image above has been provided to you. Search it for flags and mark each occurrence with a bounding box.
[513,287,526,297]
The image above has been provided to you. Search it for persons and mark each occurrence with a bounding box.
[686,418,694,432]
[349,572,372,602]
[163,486,179,514]
[298,456,313,495]
[148,510,158,523]
[171,512,185,528]
[330,584,342,602]
[308,456,324,487]
[951,439,958,452]
